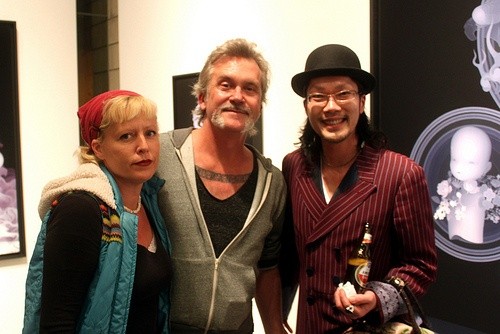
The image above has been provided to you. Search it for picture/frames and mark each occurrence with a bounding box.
[173,72,263,157]
[0,20,26,261]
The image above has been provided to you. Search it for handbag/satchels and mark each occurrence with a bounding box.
[342,276,437,334]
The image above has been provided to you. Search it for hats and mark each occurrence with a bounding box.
[291,44,377,98]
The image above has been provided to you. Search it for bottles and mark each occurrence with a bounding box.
[345,220,373,295]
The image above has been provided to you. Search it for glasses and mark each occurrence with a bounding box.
[306,90,363,104]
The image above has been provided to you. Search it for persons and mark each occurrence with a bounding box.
[153,39,292,334]
[22,89,175,334]
[278,43,441,334]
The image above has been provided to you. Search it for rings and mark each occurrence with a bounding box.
[346,305,354,314]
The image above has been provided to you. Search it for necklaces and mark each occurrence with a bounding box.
[123,194,143,215]
[321,149,358,169]
